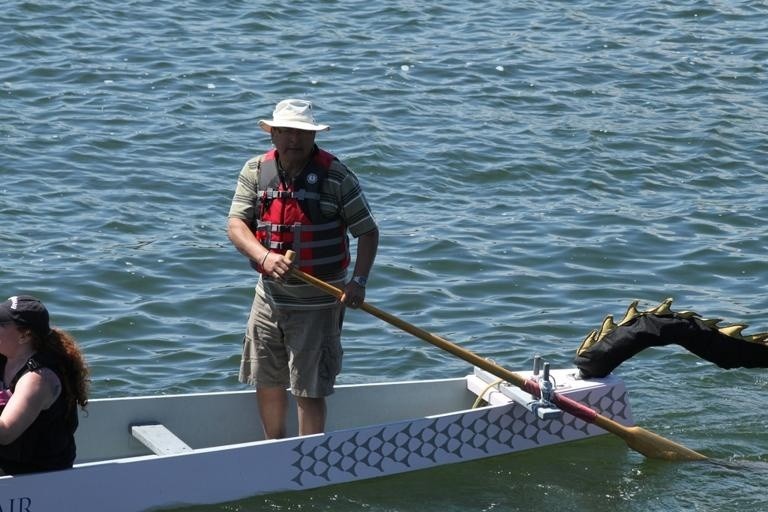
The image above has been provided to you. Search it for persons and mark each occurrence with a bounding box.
[227,99,379,440]
[0,294,89,474]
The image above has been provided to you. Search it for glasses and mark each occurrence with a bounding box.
[277,127,314,138]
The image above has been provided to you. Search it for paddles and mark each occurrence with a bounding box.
[275,251,713,461]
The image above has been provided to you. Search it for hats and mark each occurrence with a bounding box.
[257,99,330,133]
[0,295,49,323]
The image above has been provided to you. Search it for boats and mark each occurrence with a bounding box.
[0,360,637,511]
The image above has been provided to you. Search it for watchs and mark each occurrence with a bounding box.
[351,276,368,287]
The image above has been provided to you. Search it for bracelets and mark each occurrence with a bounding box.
[261,250,271,267]
